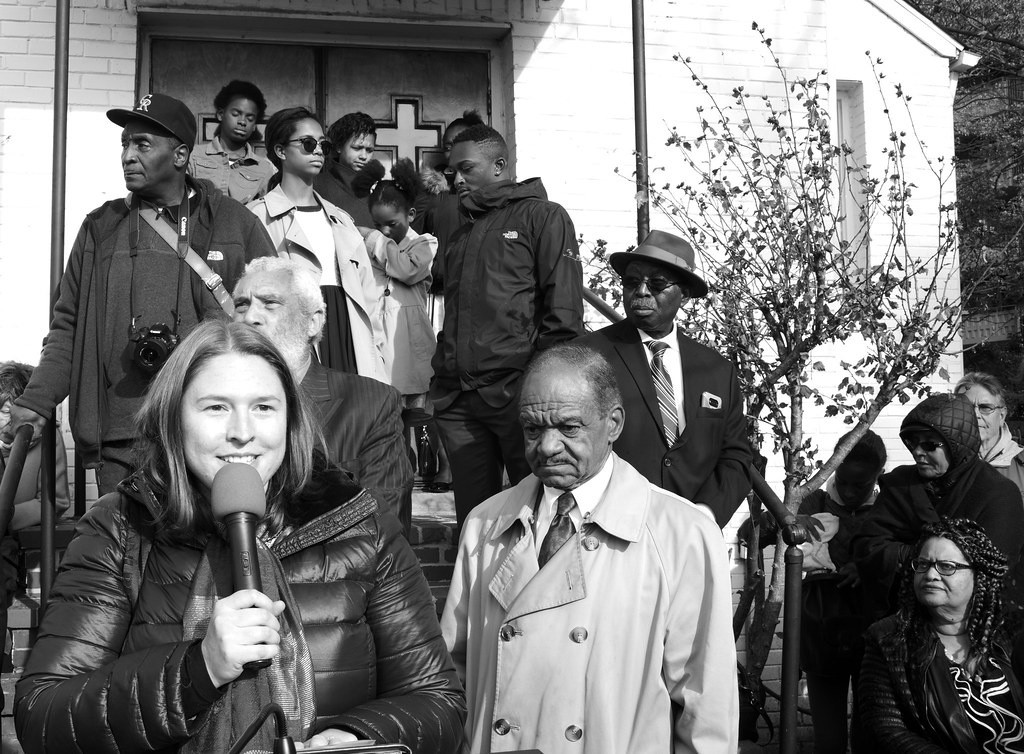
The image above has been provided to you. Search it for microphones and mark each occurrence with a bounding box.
[211,462,272,670]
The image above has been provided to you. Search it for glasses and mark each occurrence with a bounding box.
[619,277,682,294]
[284,139,333,156]
[974,404,1003,415]
[911,557,975,574]
[909,441,948,452]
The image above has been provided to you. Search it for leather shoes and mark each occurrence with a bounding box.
[428,473,454,493]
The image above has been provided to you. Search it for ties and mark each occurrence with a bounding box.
[645,340,681,448]
[538,491,578,568]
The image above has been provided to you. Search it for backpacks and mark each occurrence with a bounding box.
[738,672,773,745]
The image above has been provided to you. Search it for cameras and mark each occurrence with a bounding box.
[129,323,181,373]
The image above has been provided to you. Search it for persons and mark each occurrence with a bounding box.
[954,372,1024,503]
[442,125,585,554]
[10,93,282,500]
[239,104,390,394]
[173,81,282,209]
[552,230,756,549]
[315,109,391,233]
[228,249,408,530]
[349,157,444,463]
[438,343,740,754]
[0,360,73,712]
[410,107,488,494]
[850,393,1024,677]
[11,316,469,754]
[789,428,892,753]
[849,511,1024,754]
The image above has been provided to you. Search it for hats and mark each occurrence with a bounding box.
[610,230,707,298]
[900,425,930,439]
[108,94,197,150]
